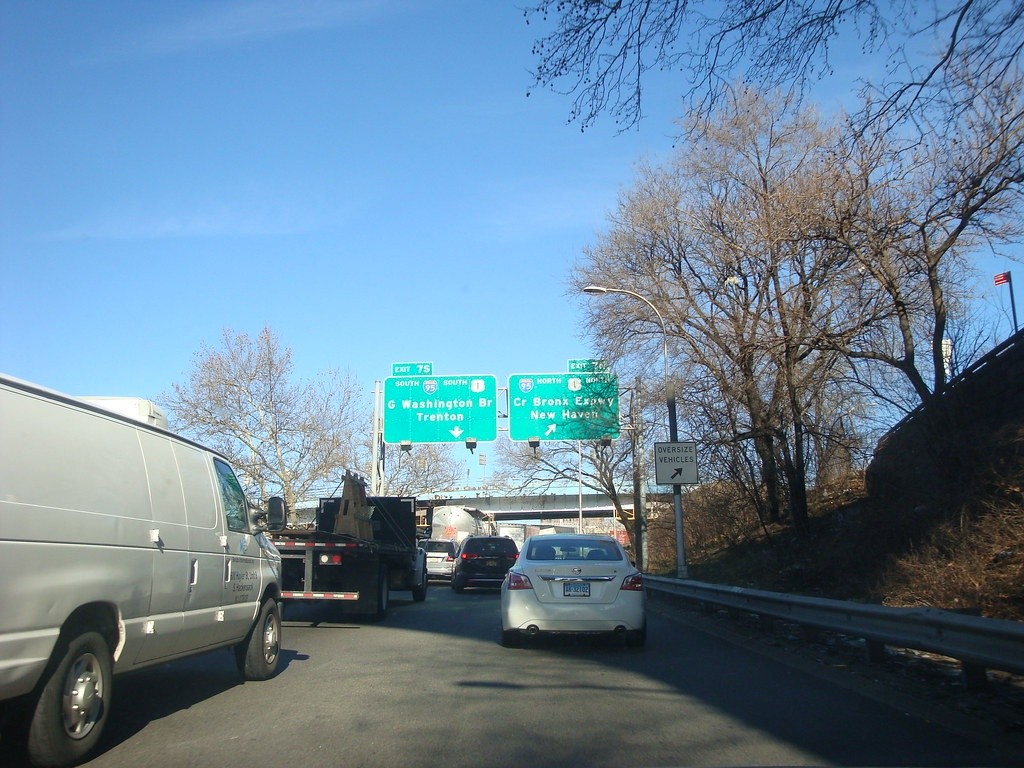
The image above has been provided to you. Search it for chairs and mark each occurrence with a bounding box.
[536,545,556,559]
[586,550,609,559]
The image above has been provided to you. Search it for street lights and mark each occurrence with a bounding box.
[581,284,687,578]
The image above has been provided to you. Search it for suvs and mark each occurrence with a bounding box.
[448,534,519,593]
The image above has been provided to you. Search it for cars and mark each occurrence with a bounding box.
[416,537,459,580]
[498,532,650,647]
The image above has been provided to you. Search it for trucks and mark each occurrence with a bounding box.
[268,495,434,624]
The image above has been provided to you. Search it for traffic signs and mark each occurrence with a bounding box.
[653,442,700,485]
[382,363,499,445]
[506,357,622,443]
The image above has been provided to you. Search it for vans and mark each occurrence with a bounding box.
[0,364,289,768]
